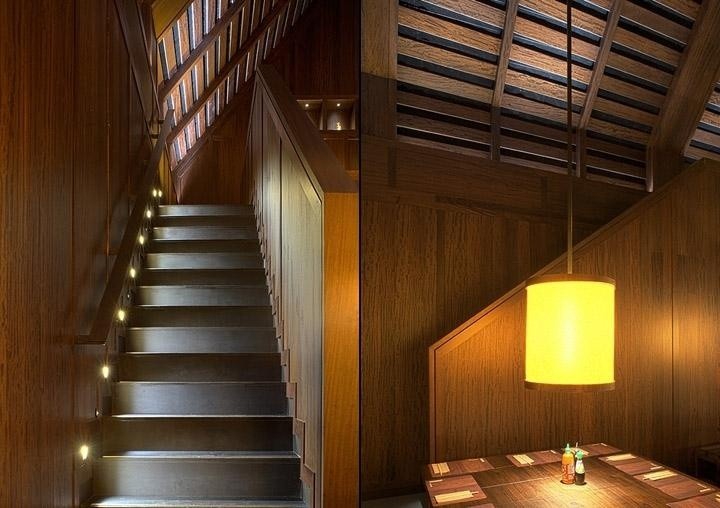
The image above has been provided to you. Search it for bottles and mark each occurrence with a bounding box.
[561,442,586,485]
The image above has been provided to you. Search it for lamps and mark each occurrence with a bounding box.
[524,3,617,394]
